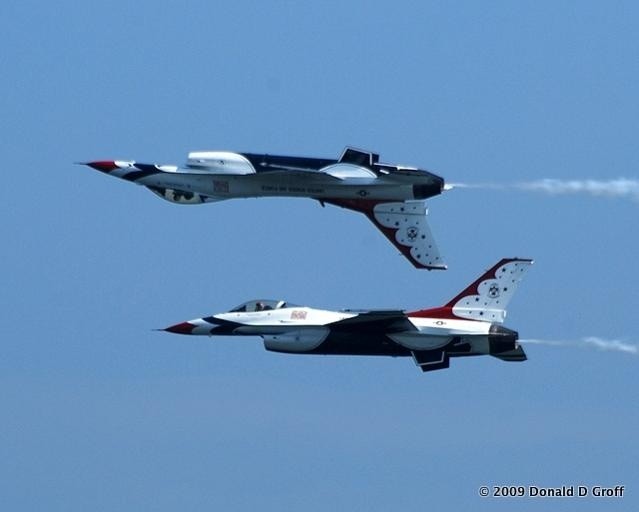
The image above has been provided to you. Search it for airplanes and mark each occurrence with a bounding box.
[76,131,452,274]
[146,254,538,376]
